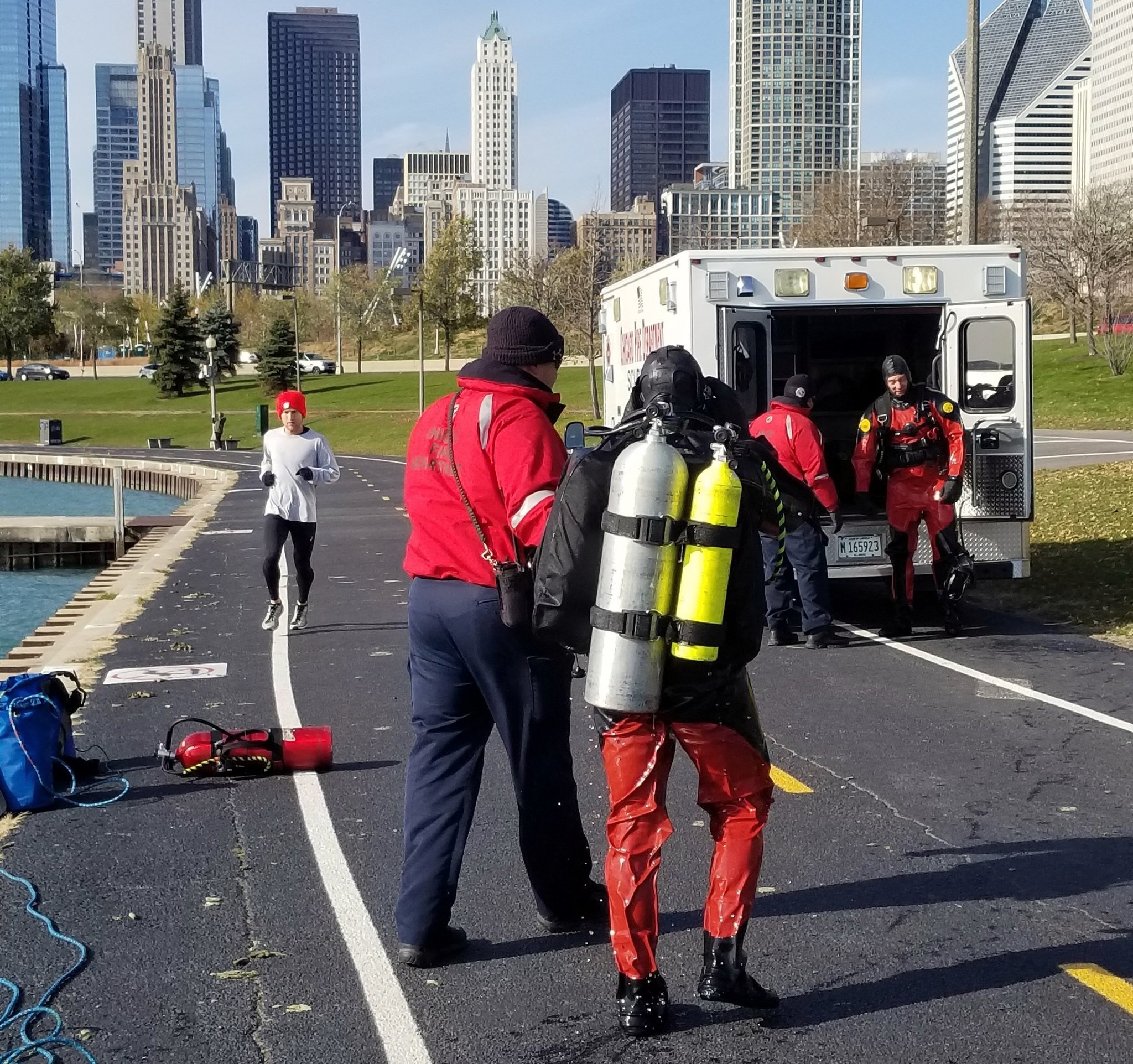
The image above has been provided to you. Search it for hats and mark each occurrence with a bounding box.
[276,391,306,419]
[482,307,564,364]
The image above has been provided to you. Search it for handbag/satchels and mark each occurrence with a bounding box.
[493,561,534,627]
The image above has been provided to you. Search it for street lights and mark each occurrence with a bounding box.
[277,294,301,391]
[336,201,352,374]
[388,287,425,418]
[70,248,85,367]
[861,216,899,247]
[135,318,140,345]
[205,334,221,450]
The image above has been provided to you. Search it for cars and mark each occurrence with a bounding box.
[16,363,69,381]
[239,350,262,364]
[293,352,336,376]
[0,370,8,381]
[138,362,163,379]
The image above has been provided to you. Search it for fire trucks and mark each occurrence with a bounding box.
[599,241,1034,619]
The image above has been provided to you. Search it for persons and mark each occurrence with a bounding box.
[394,306,610,967]
[581,345,781,1034]
[260,390,341,632]
[752,357,973,647]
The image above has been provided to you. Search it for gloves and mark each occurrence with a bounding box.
[939,481,962,504]
[262,474,274,487]
[295,467,313,481]
[829,505,843,533]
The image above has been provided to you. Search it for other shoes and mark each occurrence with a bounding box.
[619,977,669,1040]
[767,628,798,646]
[700,973,779,1009]
[398,926,468,967]
[537,882,607,933]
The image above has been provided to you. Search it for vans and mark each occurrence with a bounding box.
[1098,313,1133,334]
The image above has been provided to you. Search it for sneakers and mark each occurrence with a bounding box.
[805,627,850,649]
[945,609,962,635]
[290,604,310,629]
[878,623,911,638]
[262,599,285,631]
